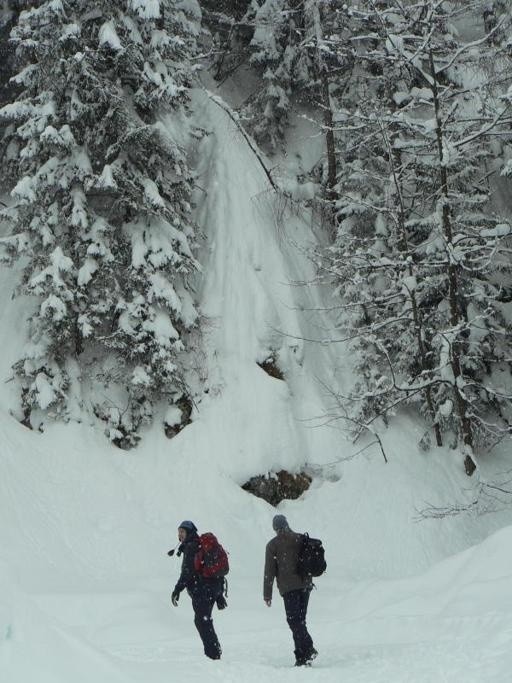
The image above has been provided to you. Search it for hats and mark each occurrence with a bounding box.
[178,519,197,531]
[272,515,289,531]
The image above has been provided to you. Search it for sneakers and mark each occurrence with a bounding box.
[292,647,318,668]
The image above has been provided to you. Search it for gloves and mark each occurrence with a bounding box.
[170,585,184,607]
[216,595,228,611]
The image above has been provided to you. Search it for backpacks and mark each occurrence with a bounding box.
[292,531,326,577]
[192,532,230,583]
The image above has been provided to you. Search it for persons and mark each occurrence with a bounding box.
[170,520,230,659]
[262,514,328,667]
